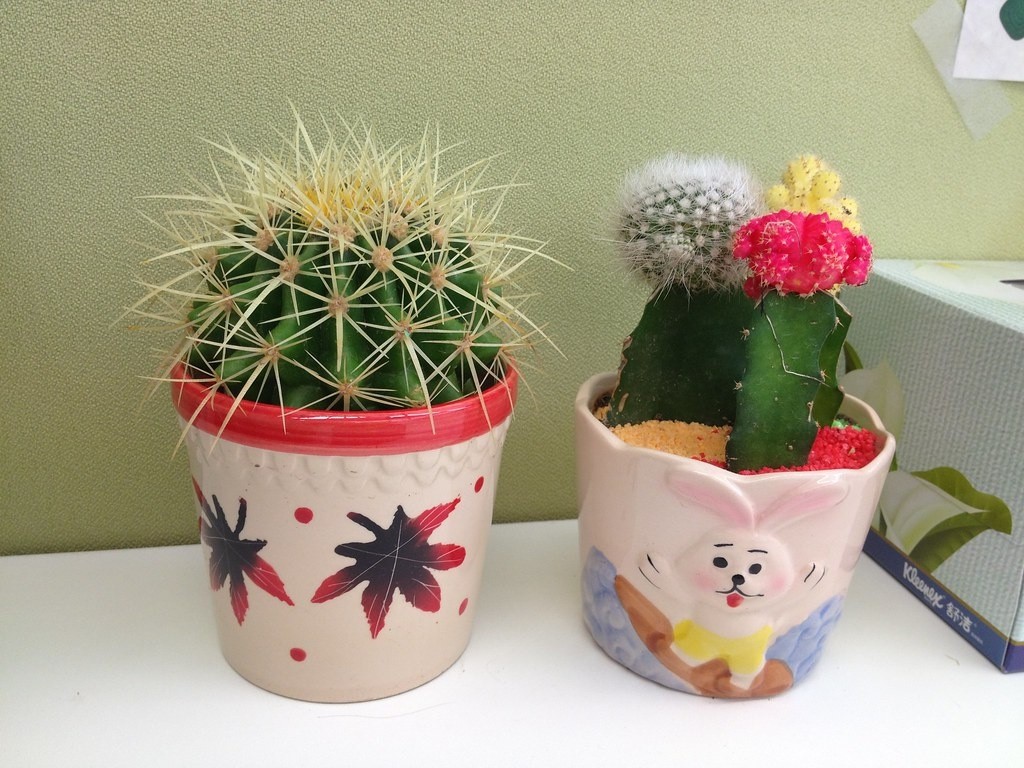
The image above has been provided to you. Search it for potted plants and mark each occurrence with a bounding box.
[574,149,897,704]
[103,91,576,706]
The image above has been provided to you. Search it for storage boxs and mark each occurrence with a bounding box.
[835,256,1024,676]
